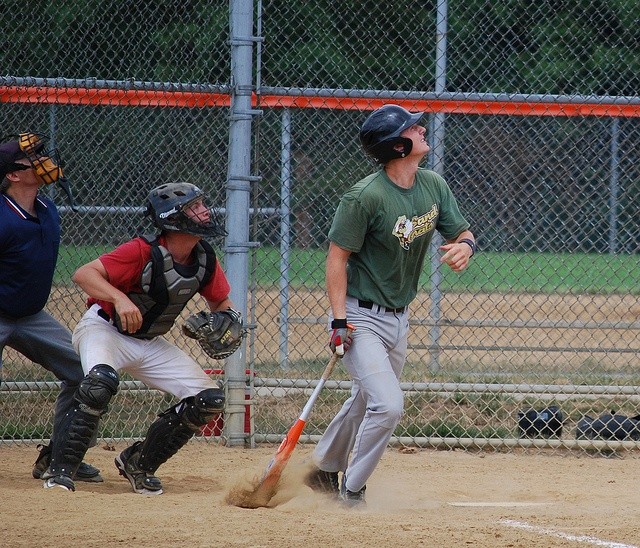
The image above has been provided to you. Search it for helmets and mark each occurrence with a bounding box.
[1,134,66,184]
[145,183,229,238]
[360,104,425,162]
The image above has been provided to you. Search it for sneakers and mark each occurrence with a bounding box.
[305,468,340,495]
[341,473,366,510]
[42,463,75,492]
[114,442,163,495]
[32,443,103,482]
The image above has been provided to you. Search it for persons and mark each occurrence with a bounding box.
[44,176,235,492]
[305,104,475,511]
[0,131,102,481]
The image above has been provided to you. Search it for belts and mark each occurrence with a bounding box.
[359,300,408,313]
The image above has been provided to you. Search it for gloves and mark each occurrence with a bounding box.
[328,318,351,357]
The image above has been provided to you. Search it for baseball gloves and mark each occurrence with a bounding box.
[183,311,247,358]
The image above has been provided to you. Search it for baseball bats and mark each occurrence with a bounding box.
[248,322,356,506]
